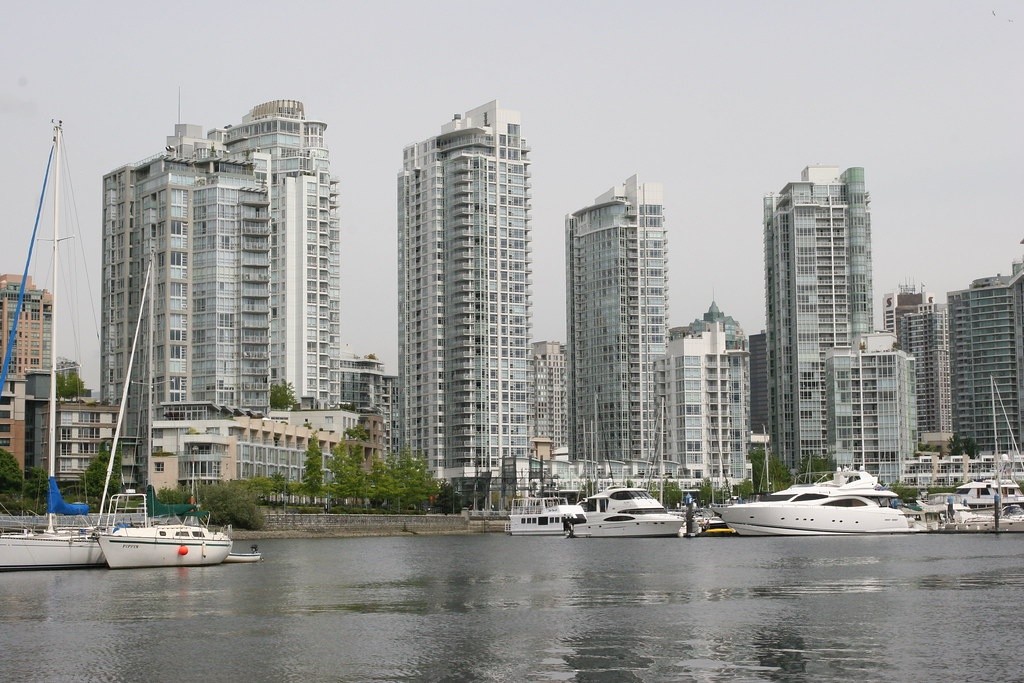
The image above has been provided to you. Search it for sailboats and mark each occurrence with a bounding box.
[0,119,111,573]
[505,369,1024,536]
[88,244,262,569]
